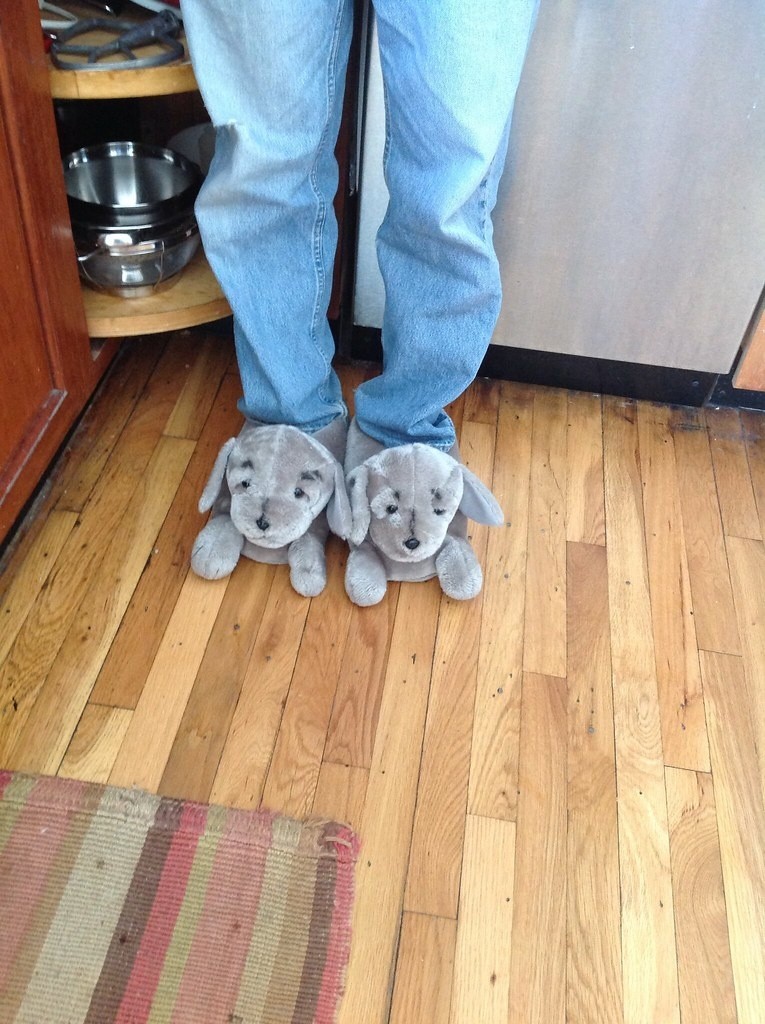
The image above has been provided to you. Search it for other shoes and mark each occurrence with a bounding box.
[343,415,503,606]
[191,412,348,596]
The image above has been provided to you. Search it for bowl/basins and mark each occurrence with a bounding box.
[63,139,201,287]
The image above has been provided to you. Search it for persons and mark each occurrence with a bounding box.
[178,0,543,608]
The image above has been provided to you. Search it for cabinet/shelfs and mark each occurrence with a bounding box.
[0,0,235,559]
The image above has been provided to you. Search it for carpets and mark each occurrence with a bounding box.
[0,769,363,1024]
